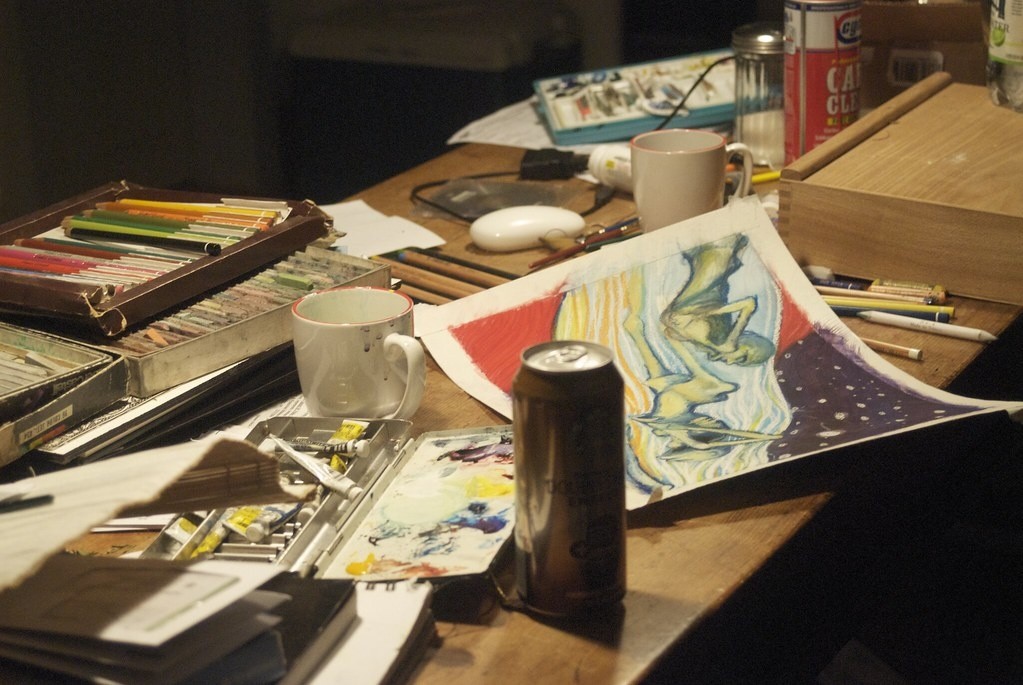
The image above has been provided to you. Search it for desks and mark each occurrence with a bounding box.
[343,71,1023,685]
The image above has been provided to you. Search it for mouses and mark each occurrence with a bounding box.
[469,205,586,252]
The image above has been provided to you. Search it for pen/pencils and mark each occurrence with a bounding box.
[810,277,954,323]
[859,337,922,361]
[528,217,643,268]
[0,245,185,297]
[13,239,192,266]
[856,310,1000,342]
[65,227,221,256]
[751,170,781,185]
[31,237,196,261]
[61,198,278,248]
[0,495,54,514]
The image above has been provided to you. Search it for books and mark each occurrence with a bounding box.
[0,551,438,685]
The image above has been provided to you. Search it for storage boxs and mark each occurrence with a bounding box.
[855,0,994,109]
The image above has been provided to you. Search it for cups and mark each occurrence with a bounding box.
[290,284,425,420]
[630,128,753,233]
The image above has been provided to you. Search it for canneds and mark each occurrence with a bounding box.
[783,0,863,166]
[512,340,628,614]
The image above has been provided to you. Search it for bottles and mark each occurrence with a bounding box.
[732,27,786,166]
[587,145,635,193]
[987,0,1022,111]
[784,0,860,169]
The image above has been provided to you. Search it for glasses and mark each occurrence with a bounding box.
[538,222,607,253]
